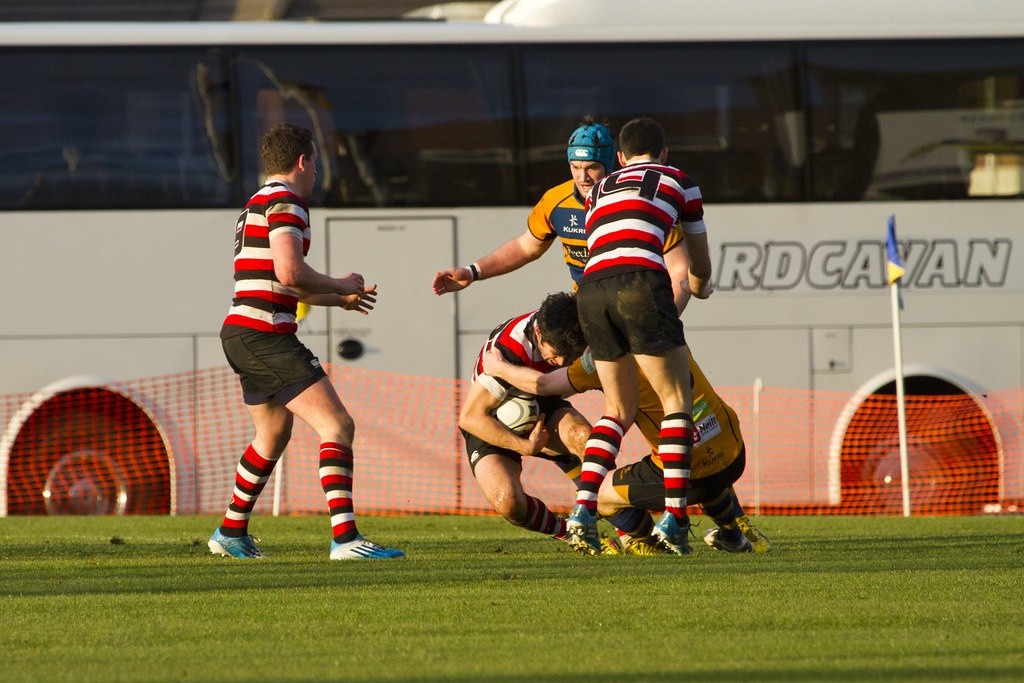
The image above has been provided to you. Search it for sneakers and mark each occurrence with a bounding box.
[330,535,405,559]
[208,527,268,558]
[567,504,601,555]
[704,514,757,552]
[736,515,771,552]
[651,510,691,556]
[554,518,625,555]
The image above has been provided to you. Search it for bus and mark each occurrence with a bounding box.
[1,0,1024,519]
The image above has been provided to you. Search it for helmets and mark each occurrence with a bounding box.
[567,123,615,174]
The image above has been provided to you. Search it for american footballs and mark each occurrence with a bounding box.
[489,388,541,439]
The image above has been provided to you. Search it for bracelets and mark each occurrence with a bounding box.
[465,263,482,282]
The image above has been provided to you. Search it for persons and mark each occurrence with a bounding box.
[430,118,769,556]
[209,122,404,561]
